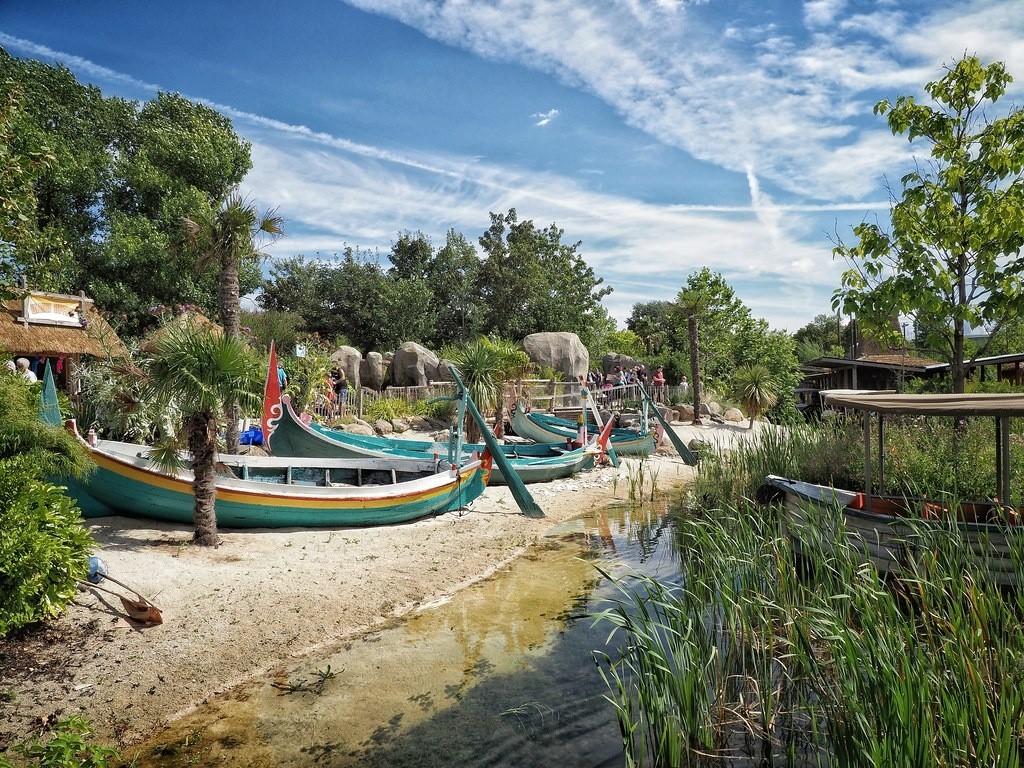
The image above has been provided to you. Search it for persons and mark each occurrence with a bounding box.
[277,359,347,420]
[587,363,688,402]
[6,358,37,383]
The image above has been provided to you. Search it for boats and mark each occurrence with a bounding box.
[297,408,615,472]
[763,388,1024,592]
[506,385,659,458]
[261,342,599,486]
[530,411,665,449]
[38,356,506,531]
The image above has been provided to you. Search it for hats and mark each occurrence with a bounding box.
[658,365,663,368]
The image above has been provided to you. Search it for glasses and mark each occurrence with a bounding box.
[15,364,23,369]
